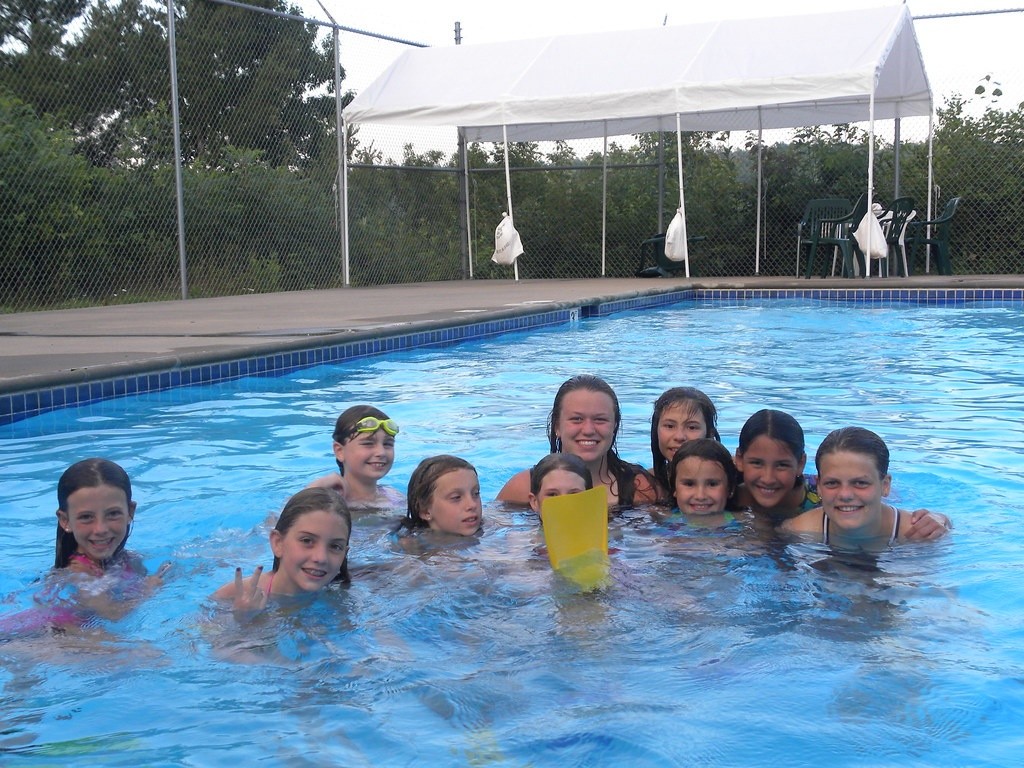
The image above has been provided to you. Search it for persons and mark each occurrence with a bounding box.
[528,452,592,526]
[307,403,396,500]
[206,484,351,620]
[52,456,137,570]
[494,373,669,510]
[670,438,739,516]
[646,387,719,491]
[780,426,925,542]
[407,455,483,536]
[730,407,953,539]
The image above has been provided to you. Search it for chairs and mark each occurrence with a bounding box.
[904,197,963,276]
[876,198,914,278]
[820,221,861,278]
[875,209,917,278]
[634,234,706,278]
[807,192,866,279]
[795,198,851,279]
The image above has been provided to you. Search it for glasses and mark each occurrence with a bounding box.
[343,417,399,438]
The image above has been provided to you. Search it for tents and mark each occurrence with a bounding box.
[335,0,936,290]
